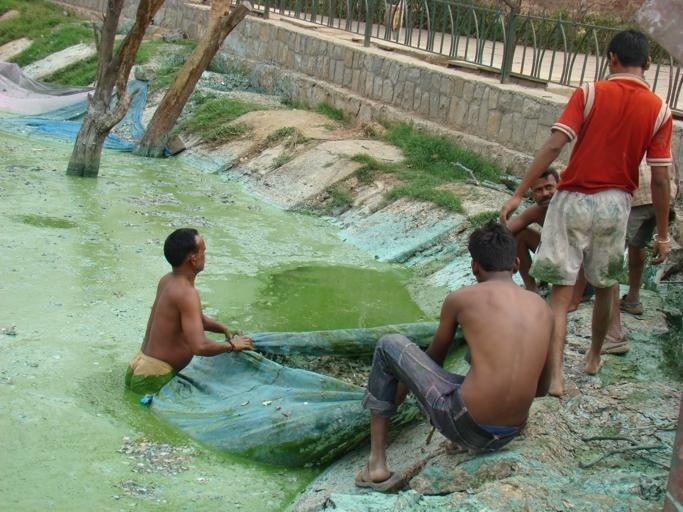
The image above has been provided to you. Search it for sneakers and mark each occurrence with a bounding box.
[620,295,643,315]
[578,334,630,353]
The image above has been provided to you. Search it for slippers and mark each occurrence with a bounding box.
[355,466,401,492]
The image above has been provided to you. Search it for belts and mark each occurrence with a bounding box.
[452,390,491,438]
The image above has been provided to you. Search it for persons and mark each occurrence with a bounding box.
[498,166,599,314]
[352,219,557,492]
[617,133,678,317]
[123,224,259,397]
[491,24,676,380]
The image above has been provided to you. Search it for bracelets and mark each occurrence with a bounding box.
[224,338,236,355]
[650,233,673,244]
